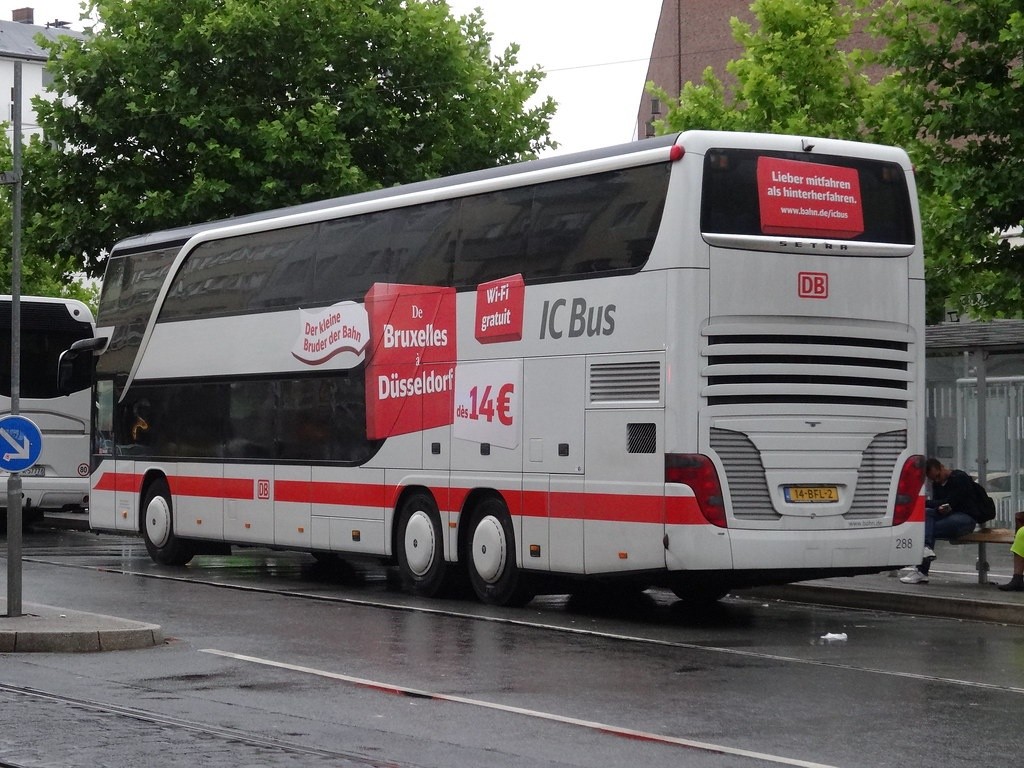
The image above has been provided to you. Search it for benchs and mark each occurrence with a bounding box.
[950,529,1016,544]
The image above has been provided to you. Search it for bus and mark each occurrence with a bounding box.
[55,129,925,610]
[0,295,96,533]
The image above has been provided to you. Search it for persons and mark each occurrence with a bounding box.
[997,526,1024,591]
[900,458,979,584]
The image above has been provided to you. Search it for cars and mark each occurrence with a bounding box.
[968,471,1024,530]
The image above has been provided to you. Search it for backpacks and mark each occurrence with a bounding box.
[946,472,996,524]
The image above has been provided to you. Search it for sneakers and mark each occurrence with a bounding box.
[923,547,937,561]
[900,568,929,583]
[998,574,1024,592]
